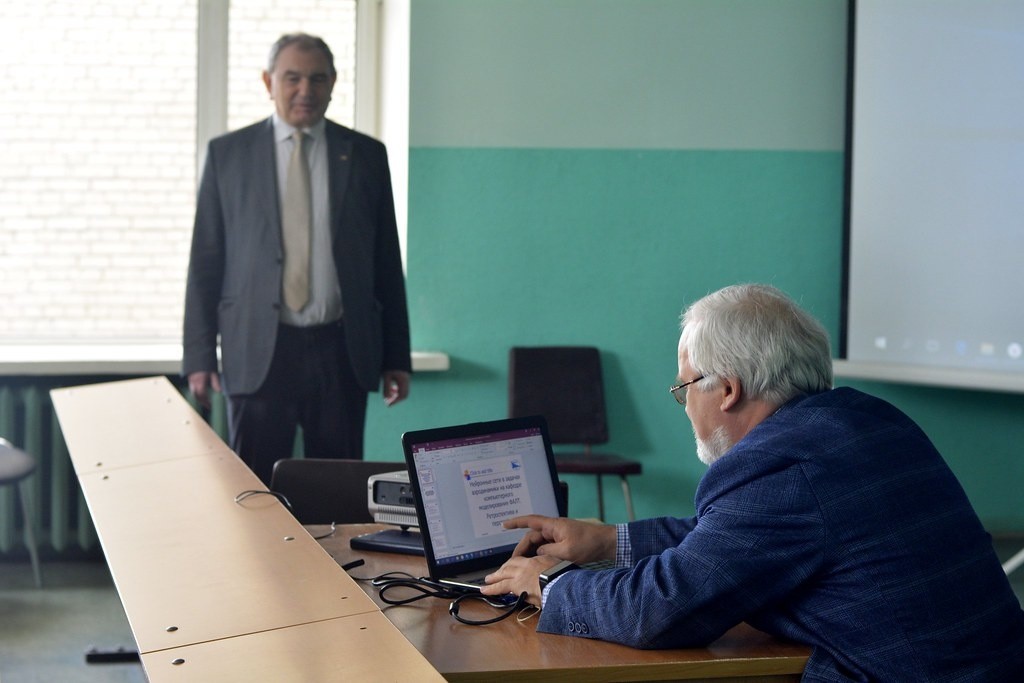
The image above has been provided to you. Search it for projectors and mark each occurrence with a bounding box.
[367,470,420,530]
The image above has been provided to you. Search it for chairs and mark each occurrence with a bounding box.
[0,438,43,591]
[273,457,407,525]
[508,347,643,522]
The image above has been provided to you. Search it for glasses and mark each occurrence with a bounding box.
[669,371,717,406]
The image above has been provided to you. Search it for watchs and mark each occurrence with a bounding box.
[536,561,582,596]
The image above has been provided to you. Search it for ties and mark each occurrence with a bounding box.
[280,131,311,314]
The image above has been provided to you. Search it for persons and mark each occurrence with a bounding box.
[478,284,1024,683]
[180,33,413,487]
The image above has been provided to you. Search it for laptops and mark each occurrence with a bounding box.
[400,415,616,604]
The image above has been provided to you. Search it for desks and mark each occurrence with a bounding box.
[299,517,813,683]
